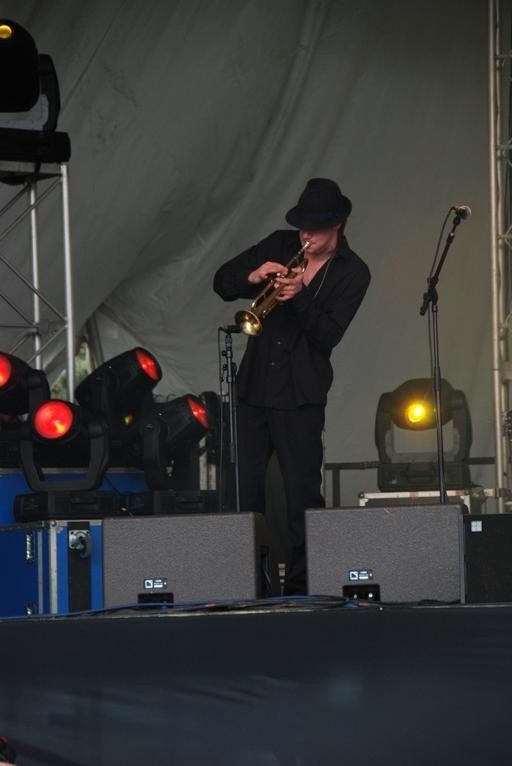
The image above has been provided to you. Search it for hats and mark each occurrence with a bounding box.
[285,175,353,232]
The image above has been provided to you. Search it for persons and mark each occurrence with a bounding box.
[213,177,370,596]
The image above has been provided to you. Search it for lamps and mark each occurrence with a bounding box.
[0,18,74,161]
[13,399,122,522]
[122,394,211,514]
[74,347,162,468]
[0,352,51,467]
[375,379,472,491]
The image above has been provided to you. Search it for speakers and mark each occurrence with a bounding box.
[304,503,468,605]
[101,511,276,607]
[463,513,512,603]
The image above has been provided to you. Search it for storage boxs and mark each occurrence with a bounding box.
[358,489,471,513]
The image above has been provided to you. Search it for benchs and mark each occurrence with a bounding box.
[324,457,495,507]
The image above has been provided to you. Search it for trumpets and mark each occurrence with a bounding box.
[234,240,311,337]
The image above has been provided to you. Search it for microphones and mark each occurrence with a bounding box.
[456,206,471,220]
